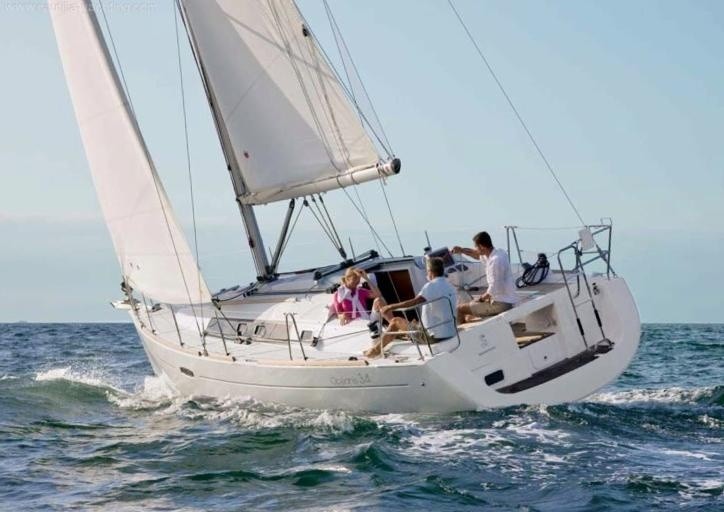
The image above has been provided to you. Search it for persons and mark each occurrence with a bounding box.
[332,266,417,339]
[361,257,460,358]
[448,231,521,323]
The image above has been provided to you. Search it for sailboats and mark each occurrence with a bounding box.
[41,0,657,420]
